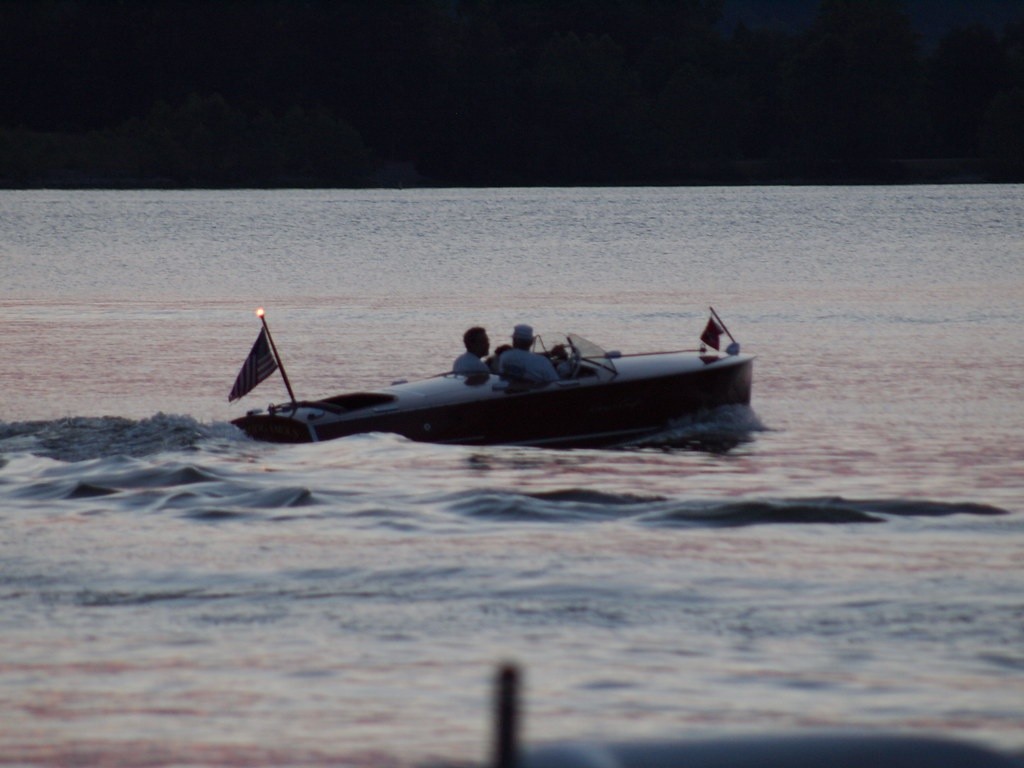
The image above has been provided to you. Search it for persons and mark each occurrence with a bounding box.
[499,324,565,385]
[453,327,511,374]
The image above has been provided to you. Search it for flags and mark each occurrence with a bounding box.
[701,318,723,351]
[227,328,279,401]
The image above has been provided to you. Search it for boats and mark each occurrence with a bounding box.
[229,332,759,450]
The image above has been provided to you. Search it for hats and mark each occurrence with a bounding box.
[511,324,536,340]
